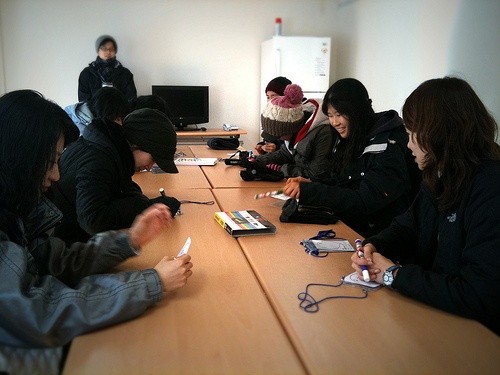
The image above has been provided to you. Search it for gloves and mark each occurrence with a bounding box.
[158,196,181,217]
[266,163,282,171]
[279,198,338,225]
[248,156,256,162]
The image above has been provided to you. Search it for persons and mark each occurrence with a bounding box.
[351,77,500,338]
[283,77,426,240]
[238,77,339,182]
[43,87,180,245]
[77,34,137,103]
[0,89,193,375]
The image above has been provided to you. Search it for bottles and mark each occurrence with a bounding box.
[275,17,281,35]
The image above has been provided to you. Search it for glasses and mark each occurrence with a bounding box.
[99,48,115,51]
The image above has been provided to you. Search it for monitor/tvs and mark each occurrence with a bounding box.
[152,85,209,131]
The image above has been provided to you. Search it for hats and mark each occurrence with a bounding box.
[95,35,117,54]
[123,108,179,173]
[261,84,305,136]
[265,77,292,96]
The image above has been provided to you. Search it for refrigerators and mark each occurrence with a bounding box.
[261,36,330,143]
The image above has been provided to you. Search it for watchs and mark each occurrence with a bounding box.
[382,265,402,290]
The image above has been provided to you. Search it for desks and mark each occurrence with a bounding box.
[62,143,500,375]
[176,127,247,145]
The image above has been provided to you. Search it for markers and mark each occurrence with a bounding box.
[254,189,284,201]
[355,239,370,282]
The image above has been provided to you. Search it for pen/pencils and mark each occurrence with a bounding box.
[160,188,165,197]
